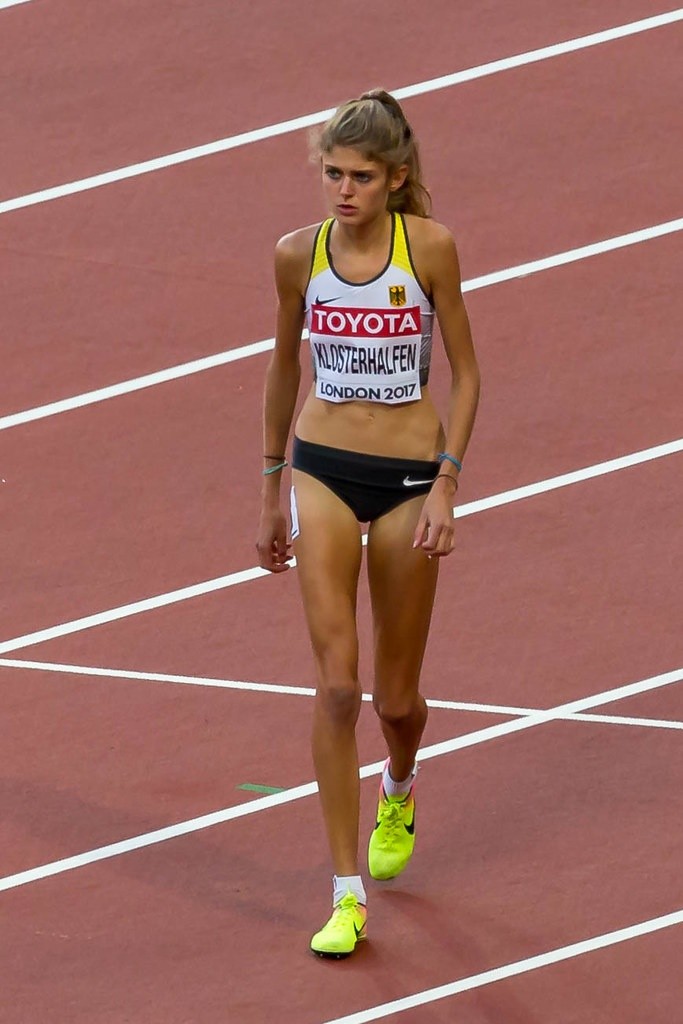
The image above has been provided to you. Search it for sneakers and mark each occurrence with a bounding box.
[367,753,418,880]
[310,894,370,960]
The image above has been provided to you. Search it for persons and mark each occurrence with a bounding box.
[247,87,481,958]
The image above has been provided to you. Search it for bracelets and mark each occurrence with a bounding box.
[259,461,288,476]
[434,448,464,470]
[431,473,461,490]
[258,453,287,462]
